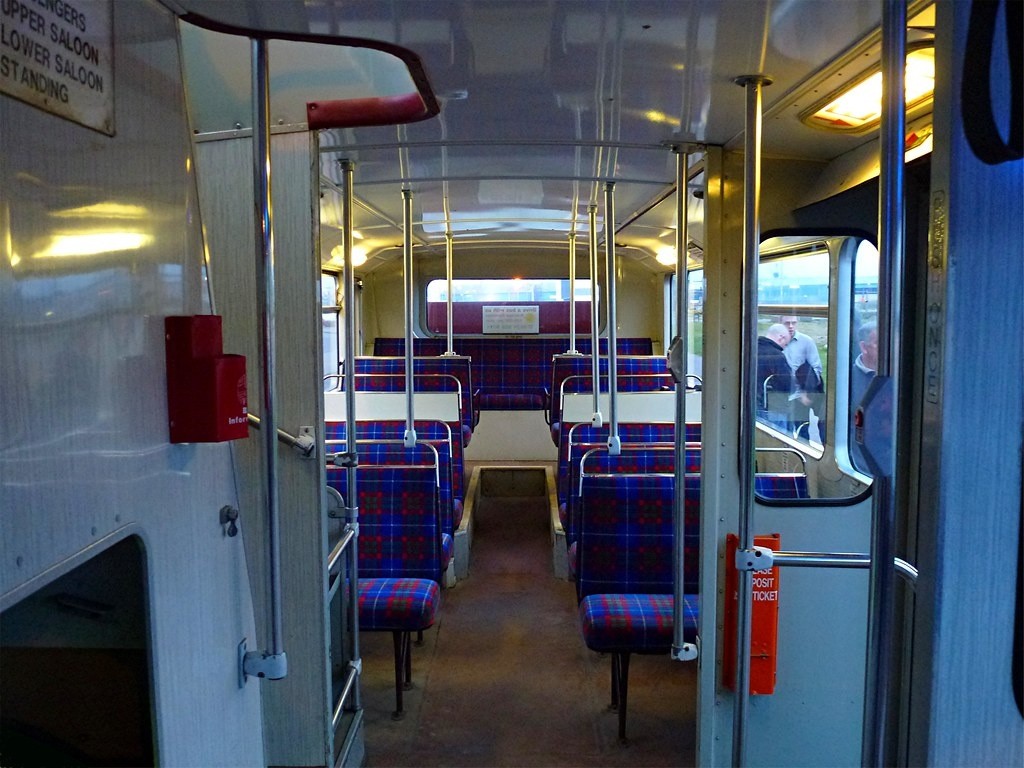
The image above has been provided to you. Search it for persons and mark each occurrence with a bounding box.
[758,323,792,430]
[779,315,823,447]
[853,321,880,478]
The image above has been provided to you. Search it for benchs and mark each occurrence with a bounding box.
[763,372,827,422]
[374,338,653,461]
[324,356,481,721]
[551,353,809,749]
